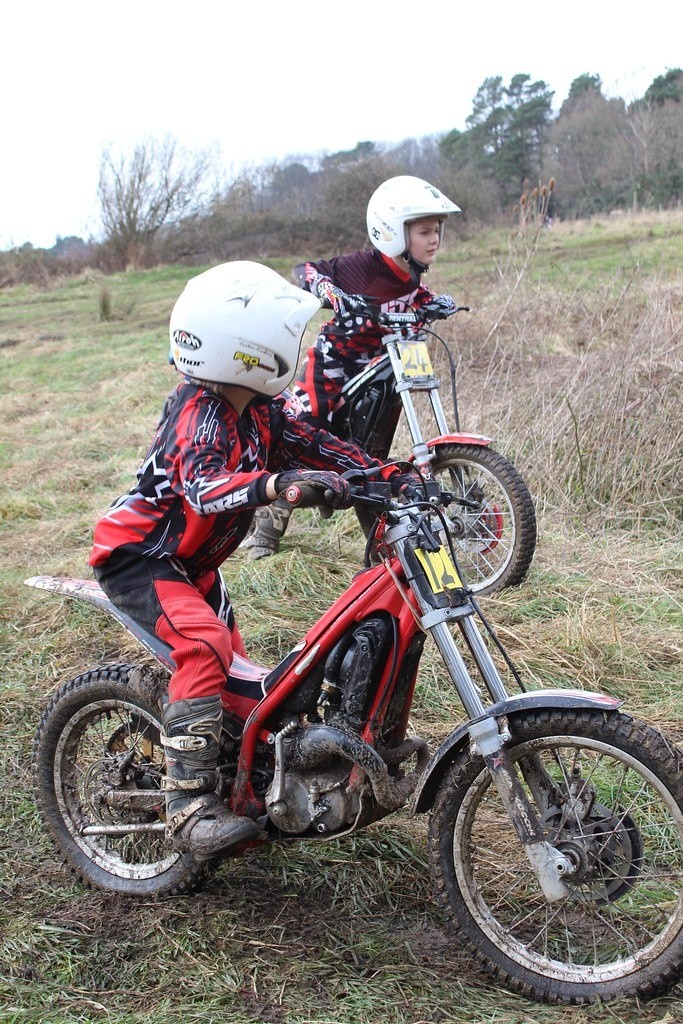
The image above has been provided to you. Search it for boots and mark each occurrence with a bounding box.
[248,495,289,560]
[157,696,259,857]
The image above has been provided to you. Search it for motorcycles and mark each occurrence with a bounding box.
[24,449,683,1005]
[272,294,538,597]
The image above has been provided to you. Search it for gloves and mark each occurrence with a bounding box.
[275,470,354,518]
[425,294,466,322]
[316,280,360,321]
[388,472,426,507]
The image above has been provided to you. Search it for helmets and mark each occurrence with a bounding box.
[364,178,465,258]
[169,258,322,398]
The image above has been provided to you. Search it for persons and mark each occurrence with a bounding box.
[91,260,436,855]
[249,175,464,561]
